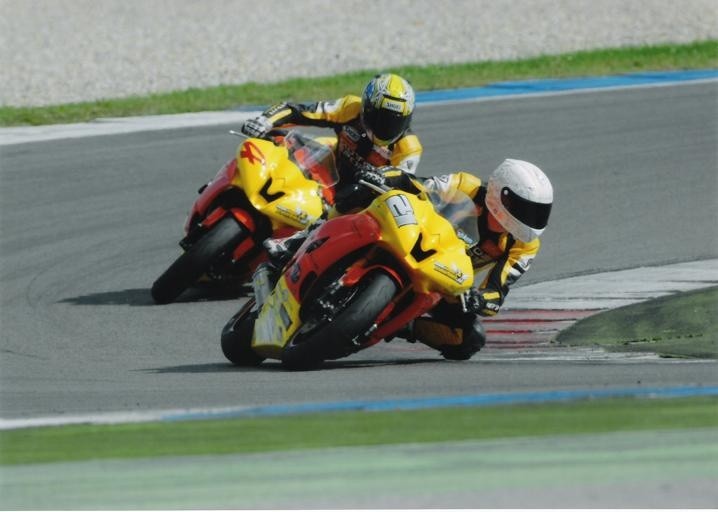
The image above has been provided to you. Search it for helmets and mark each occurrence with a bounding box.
[485,159,554,244]
[359,74,415,146]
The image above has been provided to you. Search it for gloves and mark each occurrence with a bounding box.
[354,162,386,187]
[459,289,486,314]
[241,117,270,138]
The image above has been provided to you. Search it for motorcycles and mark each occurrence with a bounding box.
[148,113,384,306]
[215,173,482,371]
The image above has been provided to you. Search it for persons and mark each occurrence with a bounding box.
[260,157,556,362]
[240,73,423,195]
